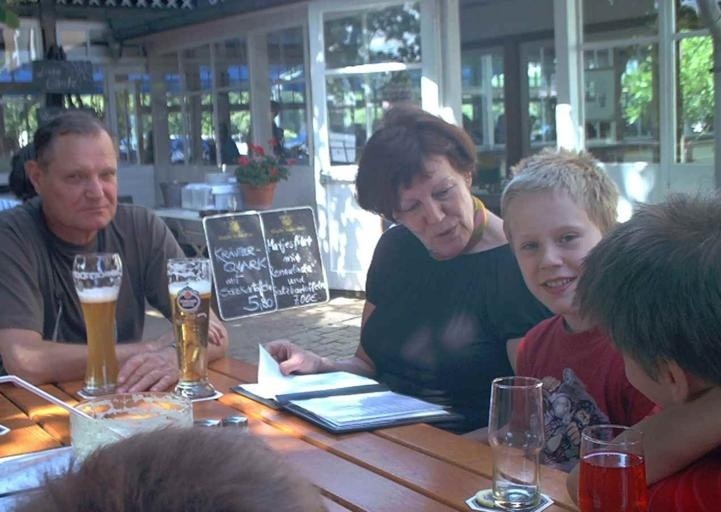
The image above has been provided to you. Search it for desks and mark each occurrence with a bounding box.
[150,208,241,259]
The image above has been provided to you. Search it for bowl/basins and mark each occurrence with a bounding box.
[71,390,195,450]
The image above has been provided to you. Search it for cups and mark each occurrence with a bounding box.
[486,374,546,510]
[578,424,649,511]
[166,257,217,398]
[70,252,125,394]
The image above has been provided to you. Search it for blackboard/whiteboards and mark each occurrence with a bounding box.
[201,205,330,322]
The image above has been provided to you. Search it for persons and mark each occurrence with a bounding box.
[122,98,558,169]
[259,104,559,447]
[565,193,721,510]
[0,427,331,510]
[1,112,229,394]
[498,146,667,471]
[8,140,38,201]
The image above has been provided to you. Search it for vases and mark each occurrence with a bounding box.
[238,181,278,211]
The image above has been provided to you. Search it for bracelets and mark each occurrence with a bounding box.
[168,341,180,350]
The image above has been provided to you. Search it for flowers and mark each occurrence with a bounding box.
[233,138,297,187]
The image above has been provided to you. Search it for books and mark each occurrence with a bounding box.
[231,342,466,435]
